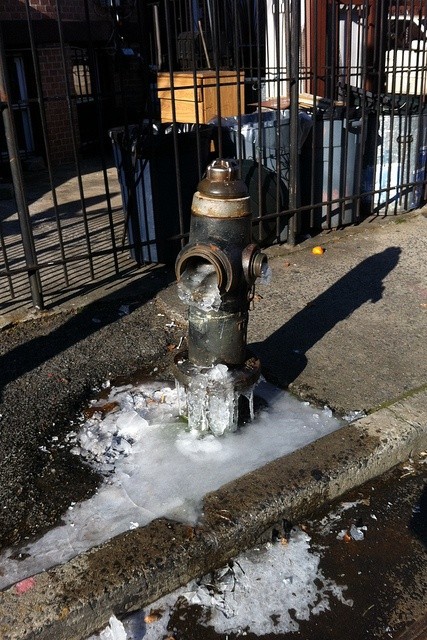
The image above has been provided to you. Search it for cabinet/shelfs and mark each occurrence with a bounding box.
[156,72,245,152]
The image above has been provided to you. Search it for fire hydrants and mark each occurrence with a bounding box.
[172,157,267,437]
